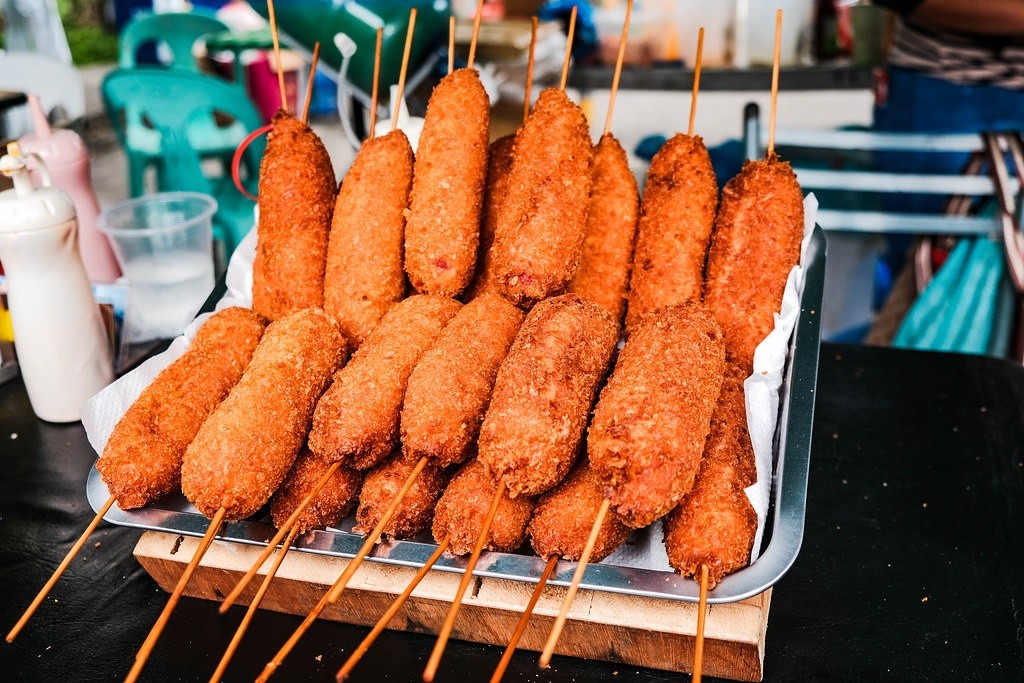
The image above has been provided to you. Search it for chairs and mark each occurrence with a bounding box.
[100,12,267,245]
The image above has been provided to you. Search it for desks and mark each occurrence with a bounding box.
[0,277,1024,683]
[527,86,876,337]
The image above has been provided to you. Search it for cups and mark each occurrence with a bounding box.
[96,190,219,337]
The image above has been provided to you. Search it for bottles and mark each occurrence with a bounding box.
[0,140,115,423]
[18,93,123,315]
[375,85,425,155]
